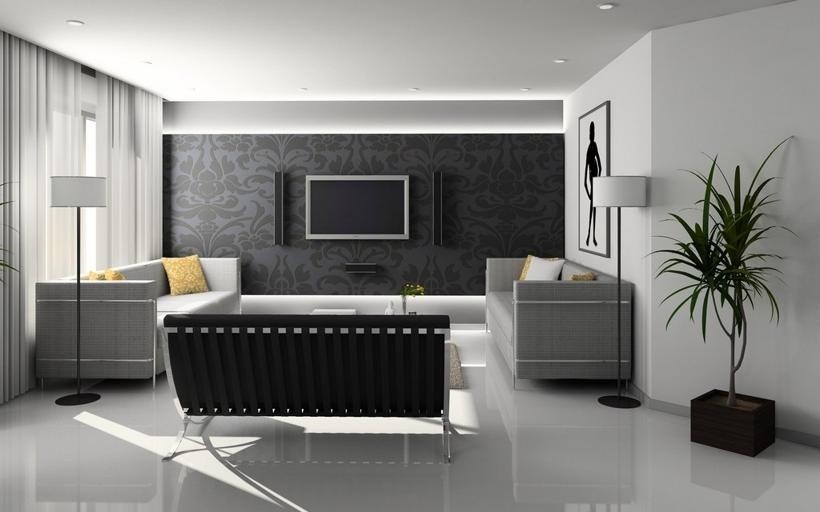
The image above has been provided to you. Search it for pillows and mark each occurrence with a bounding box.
[518,254,560,280]
[569,271,598,281]
[524,256,565,280]
[160,254,210,296]
[105,269,126,280]
[88,270,105,281]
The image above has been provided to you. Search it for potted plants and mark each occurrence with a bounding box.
[643,134,796,458]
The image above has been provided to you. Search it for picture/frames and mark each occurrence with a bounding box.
[578,100,612,259]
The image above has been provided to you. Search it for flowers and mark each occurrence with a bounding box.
[401,283,424,296]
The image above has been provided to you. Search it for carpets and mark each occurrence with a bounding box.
[446,342,466,389]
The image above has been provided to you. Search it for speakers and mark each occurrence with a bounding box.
[275,172,283,246]
[433,171,442,247]
[344,263,377,274]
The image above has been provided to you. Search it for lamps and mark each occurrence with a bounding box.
[592,173,650,409]
[50,175,109,408]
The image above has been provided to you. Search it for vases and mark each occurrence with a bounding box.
[402,296,416,314]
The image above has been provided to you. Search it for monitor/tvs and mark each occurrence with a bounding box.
[306,175,410,240]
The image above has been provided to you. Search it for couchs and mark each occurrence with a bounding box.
[36,257,243,391]
[485,257,632,389]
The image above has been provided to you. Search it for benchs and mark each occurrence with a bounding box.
[161,312,465,463]
